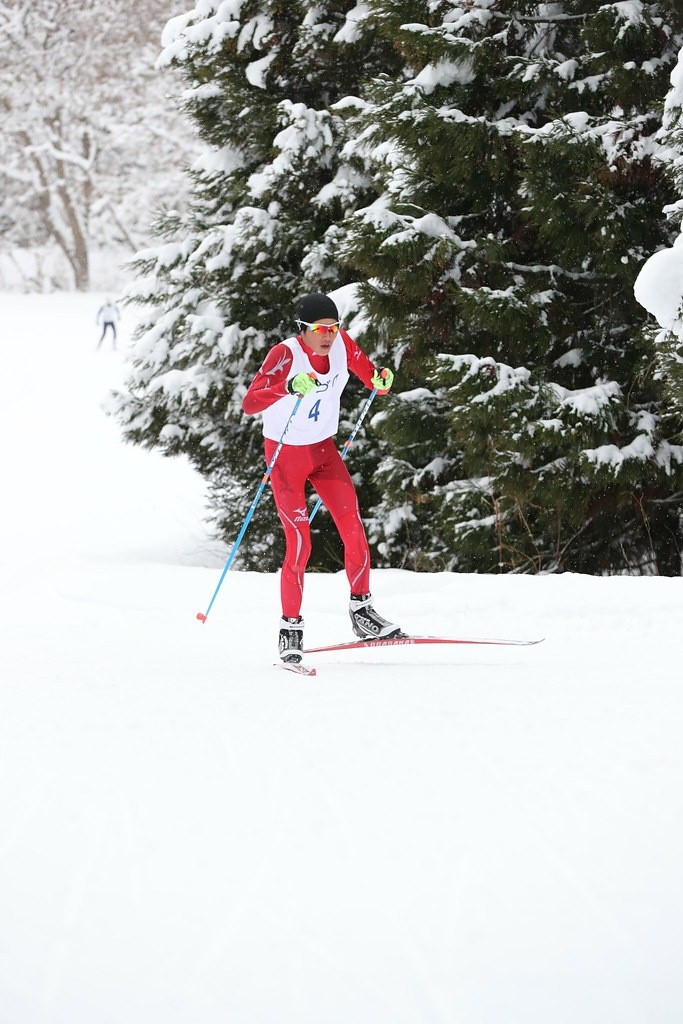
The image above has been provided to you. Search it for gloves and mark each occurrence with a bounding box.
[371,368,394,390]
[284,372,318,395]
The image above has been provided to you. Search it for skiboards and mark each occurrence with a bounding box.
[271,634,547,676]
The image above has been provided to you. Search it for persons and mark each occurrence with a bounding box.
[242,294,403,663]
[97,301,120,347]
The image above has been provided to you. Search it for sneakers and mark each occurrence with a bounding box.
[278,614,304,663]
[349,592,400,640]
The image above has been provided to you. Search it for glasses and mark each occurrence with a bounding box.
[294,320,340,334]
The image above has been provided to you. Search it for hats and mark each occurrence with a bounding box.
[295,294,338,332]
